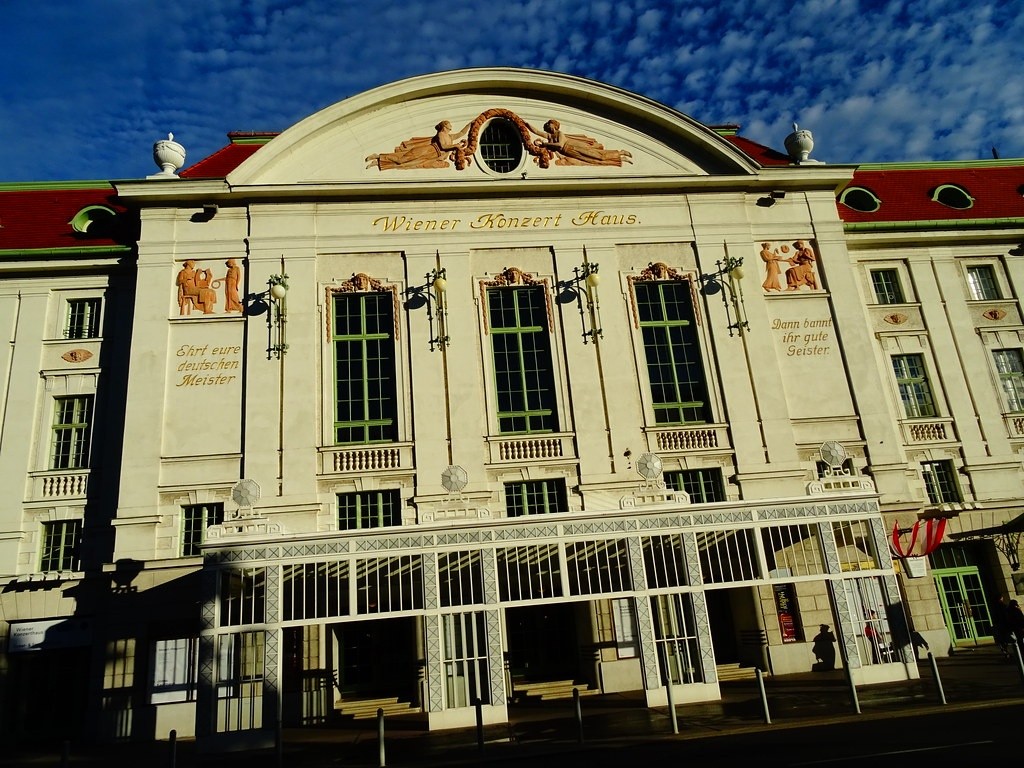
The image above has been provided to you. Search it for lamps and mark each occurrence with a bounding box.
[961,502,973,512]
[971,501,984,510]
[202,204,219,214]
[939,502,952,513]
[950,502,963,511]
[269,273,290,357]
[712,256,750,337]
[566,262,604,346]
[419,267,451,351]
[768,190,786,199]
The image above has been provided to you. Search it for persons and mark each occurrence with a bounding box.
[993,594,1024,652]
[865,610,882,665]
